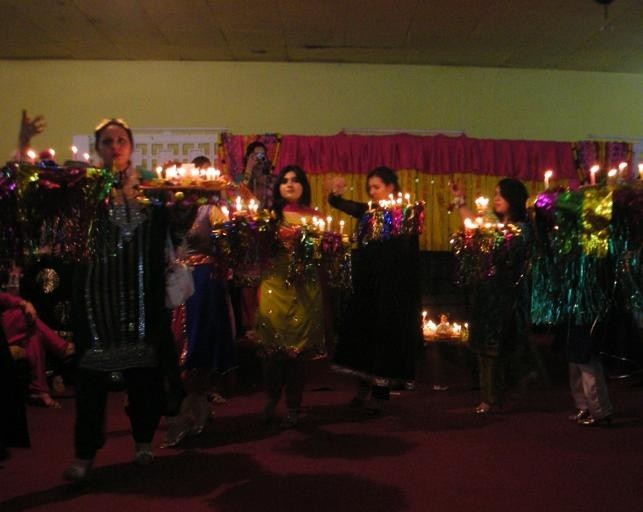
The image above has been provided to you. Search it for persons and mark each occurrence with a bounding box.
[448,177,613,427]
[0,109,429,479]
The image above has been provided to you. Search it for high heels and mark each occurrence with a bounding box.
[568,408,613,425]
[472,402,503,415]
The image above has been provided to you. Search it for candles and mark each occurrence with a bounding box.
[300,213,348,237]
[222,194,261,217]
[462,196,508,233]
[152,162,225,186]
[367,190,413,212]
[25,148,99,171]
[421,309,470,338]
[542,159,643,190]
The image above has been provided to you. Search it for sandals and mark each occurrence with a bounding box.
[32,394,61,408]
[349,385,380,416]
[160,425,203,446]
[128,435,153,464]
[279,409,298,427]
[207,392,226,404]
[63,459,90,479]
[51,376,64,392]
